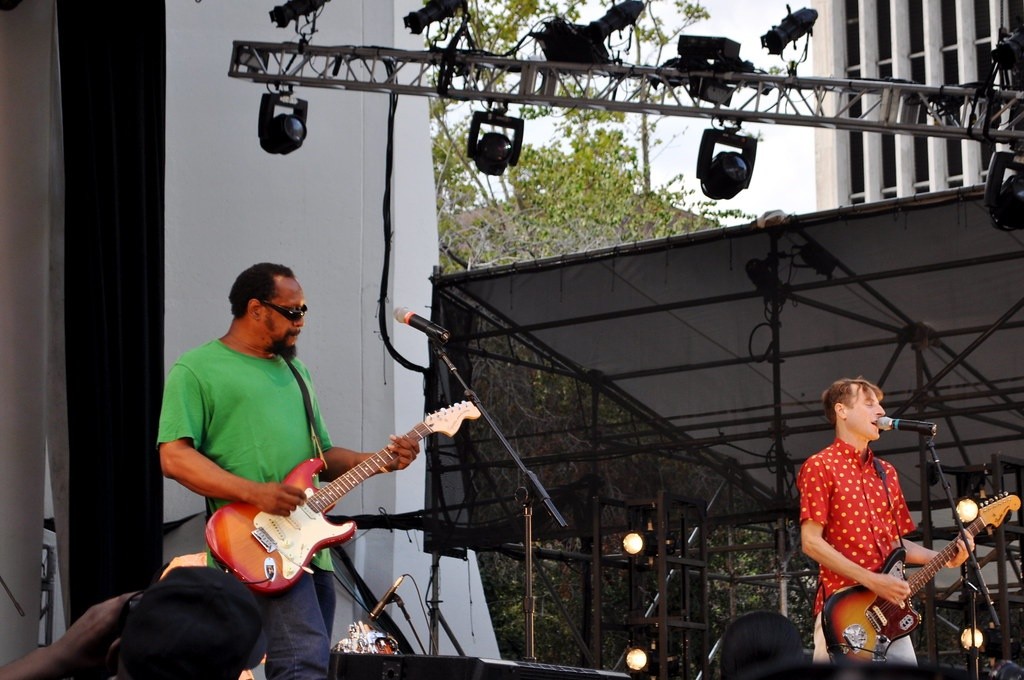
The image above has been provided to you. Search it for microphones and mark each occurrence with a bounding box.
[368,575,405,623]
[393,307,449,343]
[878,417,938,437]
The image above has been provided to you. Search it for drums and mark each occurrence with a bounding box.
[332,626,400,655]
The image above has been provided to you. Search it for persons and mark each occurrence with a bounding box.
[795,377,974,666]
[1,567,267,679]
[720,611,971,680]
[156,263,419,680]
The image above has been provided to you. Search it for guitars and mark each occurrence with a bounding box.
[203,398,481,595]
[820,490,1021,665]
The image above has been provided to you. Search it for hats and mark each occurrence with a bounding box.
[118,567,267,680]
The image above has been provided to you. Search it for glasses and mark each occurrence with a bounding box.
[260,300,307,321]
